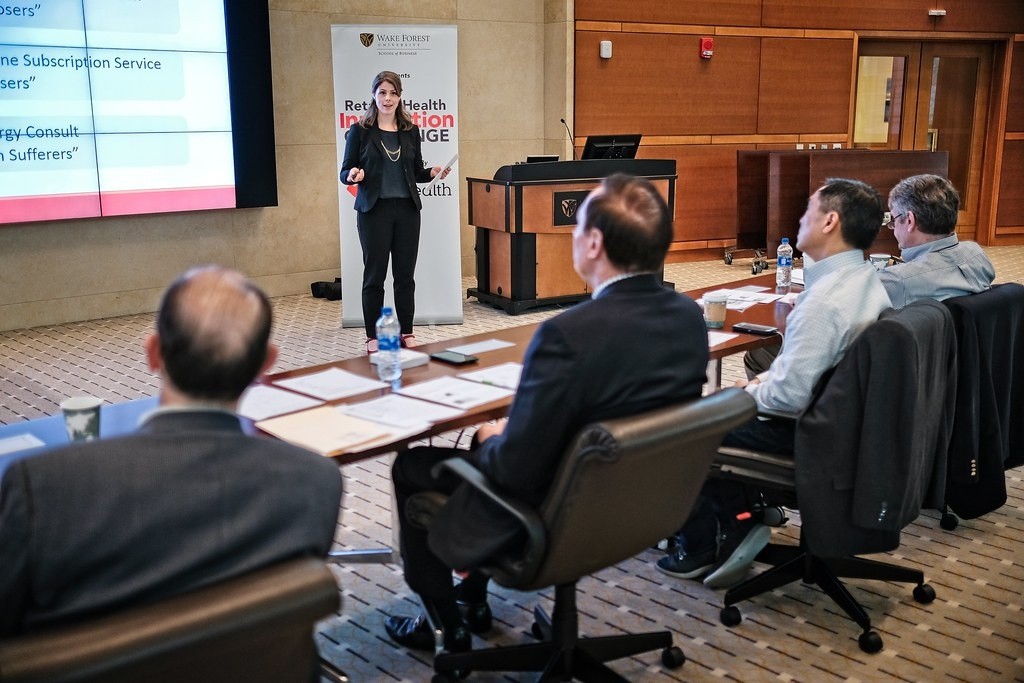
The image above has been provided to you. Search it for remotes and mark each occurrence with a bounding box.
[352,172,359,179]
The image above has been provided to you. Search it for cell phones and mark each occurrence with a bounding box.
[430,350,479,365]
[733,322,780,337]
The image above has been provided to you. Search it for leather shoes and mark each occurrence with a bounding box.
[385,613,472,653]
[456,598,492,634]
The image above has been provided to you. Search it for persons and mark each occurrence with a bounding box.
[654,175,892,580]
[873,174,998,313]
[381,172,713,653]
[0,263,343,683]
[341,71,451,355]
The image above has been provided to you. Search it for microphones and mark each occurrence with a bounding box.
[561,119,580,160]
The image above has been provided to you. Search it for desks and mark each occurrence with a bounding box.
[0,253,902,464]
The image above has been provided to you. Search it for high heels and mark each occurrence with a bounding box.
[399,333,417,348]
[366,336,378,355]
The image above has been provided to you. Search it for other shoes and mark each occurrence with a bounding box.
[656,550,715,579]
[702,523,771,590]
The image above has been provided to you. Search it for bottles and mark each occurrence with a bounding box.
[774,286,790,327]
[375,308,402,381]
[776,238,793,286]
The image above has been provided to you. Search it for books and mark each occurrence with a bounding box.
[254,403,391,457]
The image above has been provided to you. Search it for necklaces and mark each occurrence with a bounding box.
[380,138,401,163]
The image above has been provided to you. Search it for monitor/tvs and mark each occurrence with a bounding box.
[581,134,642,160]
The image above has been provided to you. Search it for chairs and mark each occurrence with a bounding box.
[939,282,1024,531]
[428,388,755,683]
[0,552,342,683]
[700,300,957,654]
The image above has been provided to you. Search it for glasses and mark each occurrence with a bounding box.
[886,212,902,230]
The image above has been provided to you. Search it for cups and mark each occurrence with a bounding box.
[60,397,104,443]
[869,254,891,271]
[702,294,727,328]
